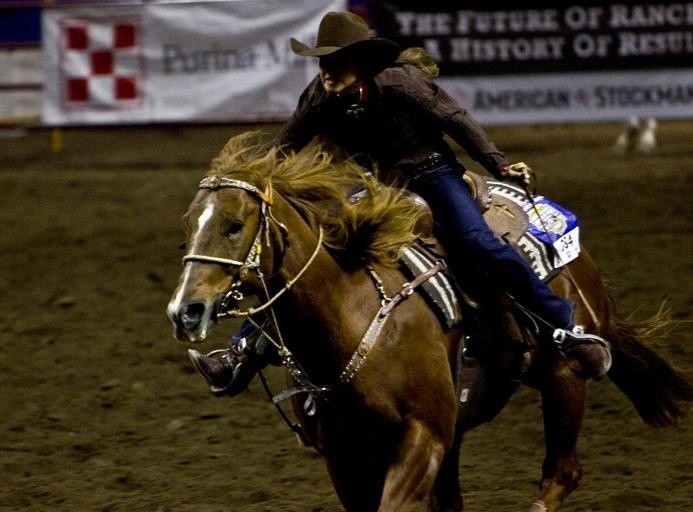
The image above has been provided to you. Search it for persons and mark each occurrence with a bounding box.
[187,9,613,394]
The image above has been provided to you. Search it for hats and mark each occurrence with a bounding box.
[290,11,397,76]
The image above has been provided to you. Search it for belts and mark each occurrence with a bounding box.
[396,154,448,182]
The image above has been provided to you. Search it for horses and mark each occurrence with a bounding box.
[167,129,692,512]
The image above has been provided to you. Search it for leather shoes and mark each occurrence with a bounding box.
[188,348,238,389]
[571,339,612,381]
